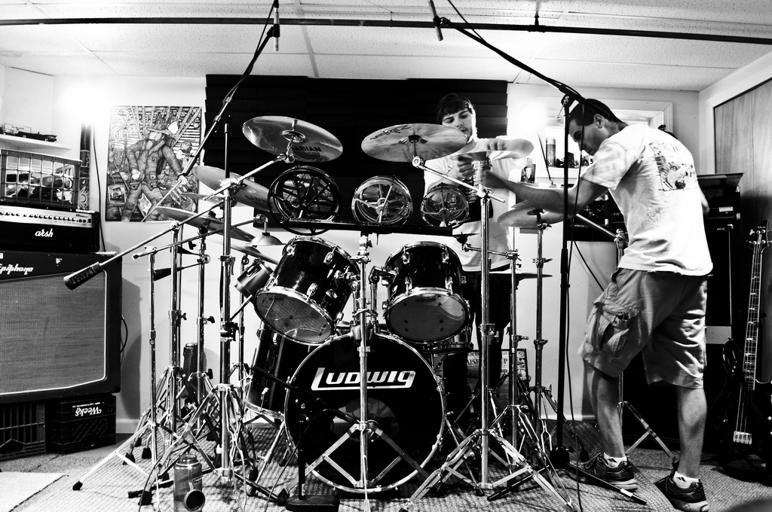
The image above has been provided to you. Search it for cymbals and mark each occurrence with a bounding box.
[361,124,468,164]
[243,115,342,164]
[154,205,255,242]
[231,244,279,266]
[497,204,564,227]
[191,165,279,214]
[489,267,551,278]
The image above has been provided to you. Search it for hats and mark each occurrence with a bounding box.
[146,118,172,136]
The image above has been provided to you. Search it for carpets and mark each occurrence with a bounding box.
[21,424,678,511]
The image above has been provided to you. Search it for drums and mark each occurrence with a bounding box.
[234,260,273,302]
[352,176,413,227]
[266,166,339,235]
[385,241,469,344]
[420,185,470,229]
[243,324,315,420]
[256,235,361,346]
[283,330,446,494]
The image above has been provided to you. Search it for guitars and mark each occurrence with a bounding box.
[708,226,771,483]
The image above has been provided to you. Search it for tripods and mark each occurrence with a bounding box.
[426,182,648,505]
[72,110,305,512]
[395,177,577,512]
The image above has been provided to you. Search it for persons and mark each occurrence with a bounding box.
[120,115,189,223]
[423,92,534,415]
[482,99,715,512]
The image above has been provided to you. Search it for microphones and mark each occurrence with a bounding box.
[273,0,280,51]
[429,0,444,41]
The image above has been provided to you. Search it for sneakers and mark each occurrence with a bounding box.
[564,452,641,490]
[654,472,710,512]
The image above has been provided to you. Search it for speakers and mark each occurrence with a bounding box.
[705,222,737,344]
[0,249,122,405]
